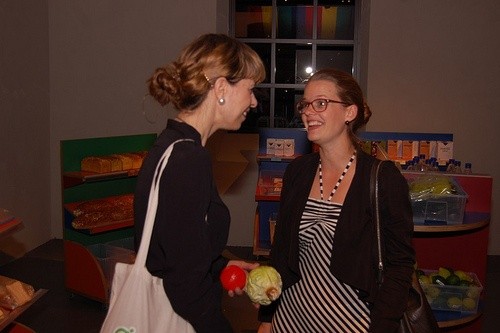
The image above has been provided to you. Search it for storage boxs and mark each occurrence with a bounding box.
[269,212,278,244]
[85,236,136,293]
[417,268,485,313]
[406,179,469,225]
[257,168,286,195]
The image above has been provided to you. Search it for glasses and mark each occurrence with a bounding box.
[296,99,348,114]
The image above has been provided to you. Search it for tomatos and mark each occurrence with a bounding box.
[220,265,246,291]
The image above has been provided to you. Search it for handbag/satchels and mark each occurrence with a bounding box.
[99,259,198,333]
[380,267,436,332]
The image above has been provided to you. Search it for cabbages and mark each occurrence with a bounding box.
[244,266,283,305]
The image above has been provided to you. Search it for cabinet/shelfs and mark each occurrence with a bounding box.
[0,216,48,333]
[58,134,154,310]
[252,129,492,333]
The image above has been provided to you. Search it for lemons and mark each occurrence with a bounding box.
[418,267,480,310]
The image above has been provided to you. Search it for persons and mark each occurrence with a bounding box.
[132,32,267,333]
[257,68,418,333]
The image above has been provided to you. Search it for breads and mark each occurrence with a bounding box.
[71,199,132,228]
[0,281,33,310]
[81,152,145,172]
